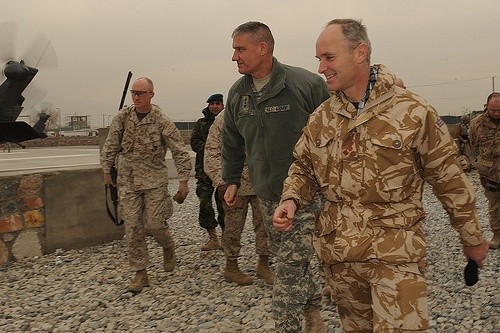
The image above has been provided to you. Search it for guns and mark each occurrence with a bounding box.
[102,72,131,226]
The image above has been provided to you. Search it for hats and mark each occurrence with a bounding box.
[207,94,223,103]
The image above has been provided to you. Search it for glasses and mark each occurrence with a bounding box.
[130,90,152,96]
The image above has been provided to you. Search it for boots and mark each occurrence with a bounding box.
[220,227,225,249]
[302,306,327,333]
[201,228,221,250]
[488,231,500,248]
[163,243,176,271]
[257,255,274,285]
[224,258,252,285]
[127,269,149,292]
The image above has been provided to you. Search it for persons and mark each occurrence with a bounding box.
[190,93,225,251]
[99,76,192,294]
[202,105,279,285]
[220,21,333,333]
[268,18,490,333]
[469,92,500,249]
